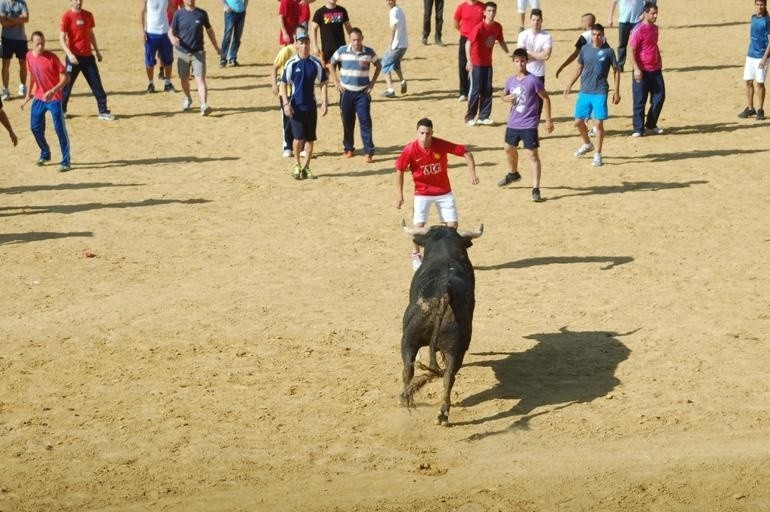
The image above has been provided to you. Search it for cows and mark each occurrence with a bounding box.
[398,219,484,428]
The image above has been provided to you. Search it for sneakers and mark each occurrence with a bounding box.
[411,251,424,270]
[631,127,664,137]
[497,171,523,188]
[458,95,467,102]
[531,187,542,203]
[422,38,444,45]
[591,153,602,166]
[36,153,72,174]
[147,58,240,117]
[737,107,764,120]
[98,113,115,120]
[381,80,407,98]
[1,85,28,100]
[574,143,593,157]
[466,118,495,127]
[282,148,375,180]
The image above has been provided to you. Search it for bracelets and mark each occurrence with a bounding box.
[51,89,55,94]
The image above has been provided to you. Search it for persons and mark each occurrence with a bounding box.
[272,0,328,180]
[378,0,408,98]
[421,0,446,47]
[330,27,381,163]
[158,0,186,80]
[140,0,176,94]
[25,30,72,172]
[219,0,249,67]
[0,94,18,147]
[311,0,352,117]
[167,0,222,117]
[0,0,29,101]
[738,0,770,120]
[395,118,479,272]
[58,0,116,121]
